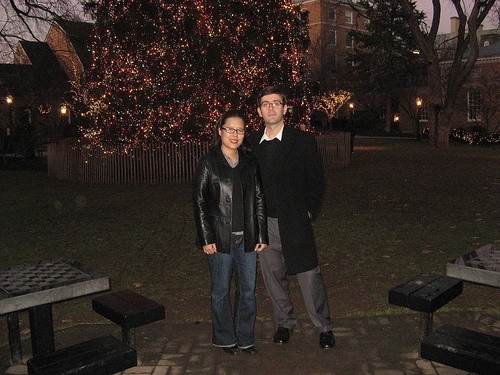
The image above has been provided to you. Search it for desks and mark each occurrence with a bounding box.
[446,239,500,287]
[0,255,110,358]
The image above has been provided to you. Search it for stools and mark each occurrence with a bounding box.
[25,335,138,375]
[92,290,166,348]
[420,325,500,375]
[388,273,463,354]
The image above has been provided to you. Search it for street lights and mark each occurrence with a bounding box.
[416,96,423,137]
[6,94,13,135]
[60,103,73,125]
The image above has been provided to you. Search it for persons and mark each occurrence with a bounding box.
[196,110,269,355]
[252,88,335,348]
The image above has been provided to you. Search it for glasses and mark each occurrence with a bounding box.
[260,102,284,107]
[221,128,246,134]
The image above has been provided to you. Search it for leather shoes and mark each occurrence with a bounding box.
[222,345,240,355]
[243,346,258,355]
[319,330,335,352]
[272,327,293,344]
